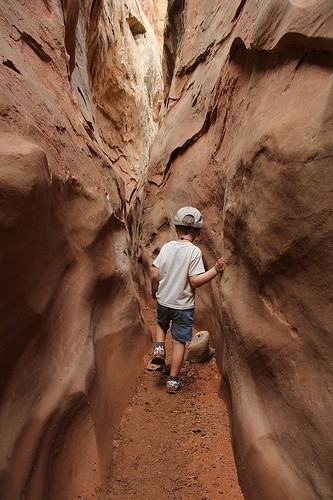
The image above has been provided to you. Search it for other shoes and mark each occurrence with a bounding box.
[152,346,165,365]
[165,379,183,393]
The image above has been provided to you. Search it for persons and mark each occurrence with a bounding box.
[151,205,225,393]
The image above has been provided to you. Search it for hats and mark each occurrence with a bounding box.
[173,206,203,228]
